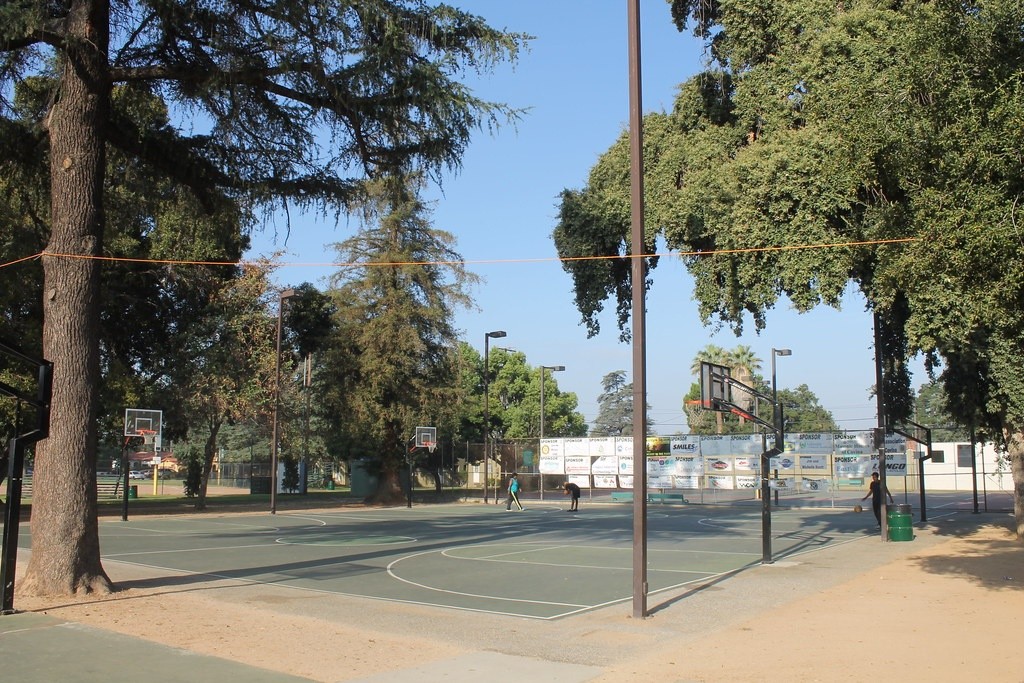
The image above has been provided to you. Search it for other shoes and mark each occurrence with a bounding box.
[519,508,524,511]
[506,510,513,511]
[876,525,881,530]
[567,509,573,511]
[574,509,577,511]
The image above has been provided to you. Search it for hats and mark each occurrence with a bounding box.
[512,472,518,475]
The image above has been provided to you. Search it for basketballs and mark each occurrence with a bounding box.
[854,505,862,513]
[563,490,569,495]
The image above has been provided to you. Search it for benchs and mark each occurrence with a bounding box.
[648,494,689,504]
[611,492,633,503]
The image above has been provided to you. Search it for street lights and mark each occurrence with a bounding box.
[539,364,567,503]
[483,330,506,503]
[269,288,303,515]
[771,346,792,505]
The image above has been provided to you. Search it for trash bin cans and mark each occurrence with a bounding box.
[128,485,137,498]
[327,480,334,490]
[887,503,915,541]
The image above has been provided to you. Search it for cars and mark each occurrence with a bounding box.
[128,468,176,480]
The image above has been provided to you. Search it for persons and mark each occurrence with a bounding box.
[862,471,894,531]
[506,472,525,511]
[563,481,580,512]
